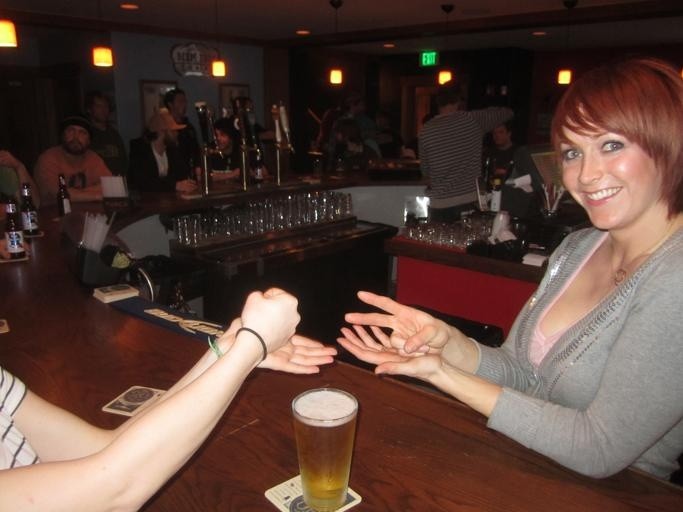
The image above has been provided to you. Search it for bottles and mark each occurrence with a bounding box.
[20,181,39,236]
[4,195,25,259]
[56,172,73,219]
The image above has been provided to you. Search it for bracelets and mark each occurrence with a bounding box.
[232,325,268,367]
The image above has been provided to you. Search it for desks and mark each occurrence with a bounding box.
[386,225,558,335]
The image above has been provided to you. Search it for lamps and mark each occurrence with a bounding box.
[0,18,19,48]
[329,68,344,85]
[91,45,114,67]
[438,69,454,86]
[557,68,573,86]
[210,59,229,78]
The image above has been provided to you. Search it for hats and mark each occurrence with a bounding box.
[60,111,90,130]
[148,108,187,133]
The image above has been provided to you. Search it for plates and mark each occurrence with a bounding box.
[23,231,44,237]
[0,239,29,262]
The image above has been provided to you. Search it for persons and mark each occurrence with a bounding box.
[164,88,204,182]
[205,116,242,182]
[33,110,112,206]
[0,288,337,511]
[335,58,683,483]
[295,94,393,171]
[417,87,513,223]
[125,110,199,194]
[84,89,127,177]
[484,124,544,220]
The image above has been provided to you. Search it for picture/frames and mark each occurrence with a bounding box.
[219,82,252,112]
[138,78,179,129]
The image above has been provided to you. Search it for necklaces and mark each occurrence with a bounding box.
[603,221,673,291]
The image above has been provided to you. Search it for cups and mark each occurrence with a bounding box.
[293,389,360,512]
